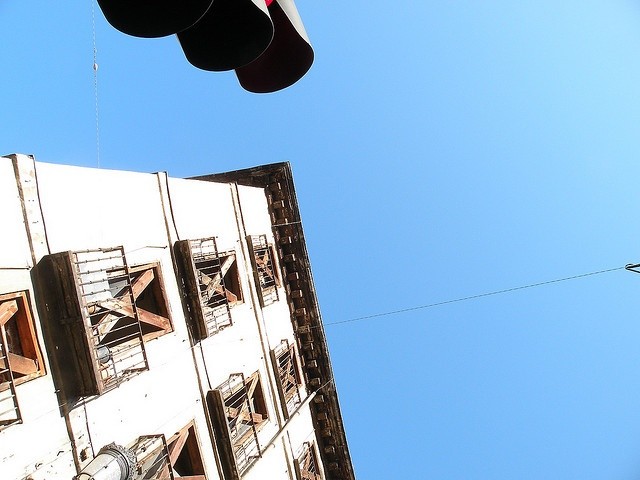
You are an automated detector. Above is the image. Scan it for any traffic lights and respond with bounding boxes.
[97,0,314,94]
